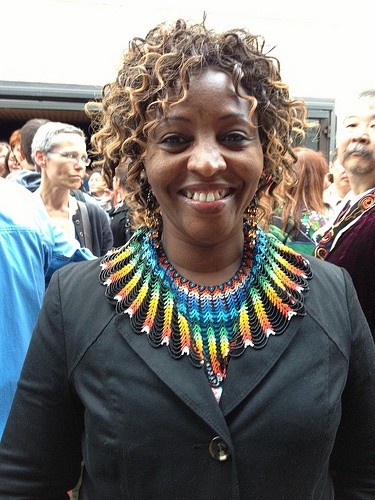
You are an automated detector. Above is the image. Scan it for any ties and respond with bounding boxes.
[315,201,349,259]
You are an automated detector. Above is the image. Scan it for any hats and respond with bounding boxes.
[10,130,22,145]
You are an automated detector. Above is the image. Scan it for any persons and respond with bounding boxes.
[0,176,99,441]
[1,20,375,500]
[312,90,375,343]
[2,119,133,258]
[268,147,355,255]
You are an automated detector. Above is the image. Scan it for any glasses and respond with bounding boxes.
[51,150,91,167]
